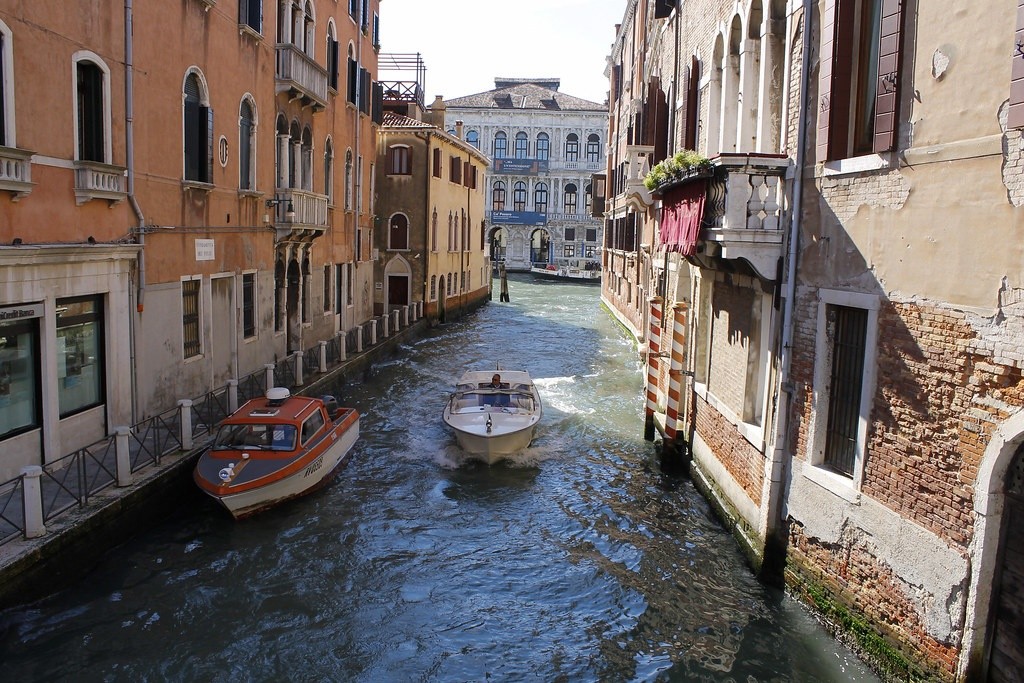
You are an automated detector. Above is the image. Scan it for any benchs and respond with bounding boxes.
[455,398,534,409]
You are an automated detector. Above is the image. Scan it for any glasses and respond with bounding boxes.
[492,378,499,381]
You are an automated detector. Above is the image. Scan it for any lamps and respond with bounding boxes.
[266,198,296,217]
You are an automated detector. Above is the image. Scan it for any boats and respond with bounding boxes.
[441,360,542,466]
[530,265,601,284]
[190,387,359,528]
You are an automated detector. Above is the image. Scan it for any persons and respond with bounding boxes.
[483,374,510,404]
